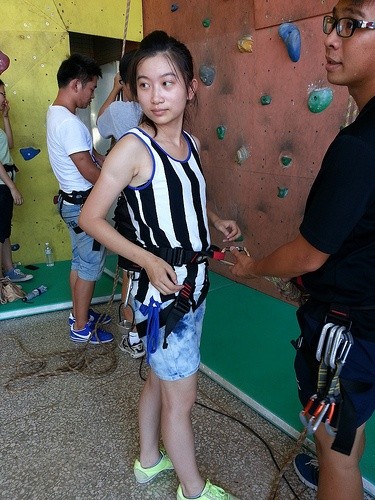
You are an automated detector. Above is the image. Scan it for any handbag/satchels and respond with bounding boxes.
[0,276,26,304]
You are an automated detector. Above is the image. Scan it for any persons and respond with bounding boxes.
[47,54,114,344]
[78,30,242,500]
[96,48,145,359]
[0,79,34,289]
[228,0,375,500]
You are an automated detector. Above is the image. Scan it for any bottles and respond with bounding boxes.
[44,242,54,267]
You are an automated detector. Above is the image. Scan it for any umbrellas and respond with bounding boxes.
[22,285,47,303]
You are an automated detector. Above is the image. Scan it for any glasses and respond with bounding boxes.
[323,16,375,38]
[119,80,124,85]
[0,92,6,98]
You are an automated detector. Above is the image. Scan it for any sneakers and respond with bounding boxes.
[117,331,145,359]
[68,308,112,327]
[3,268,33,282]
[134,442,175,484]
[69,320,114,344]
[176,479,242,500]
[293,453,319,492]
